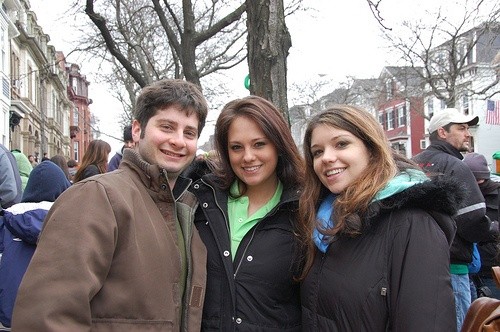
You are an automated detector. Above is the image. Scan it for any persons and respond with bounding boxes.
[0,127,137,331]
[11,79,209,332]
[292,103,472,332]
[190,96,305,332]
[402,108,500,332]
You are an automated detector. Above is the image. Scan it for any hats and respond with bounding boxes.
[461,152,490,180]
[428,108,479,134]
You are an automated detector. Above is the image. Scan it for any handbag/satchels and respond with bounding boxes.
[460,266,500,332]
